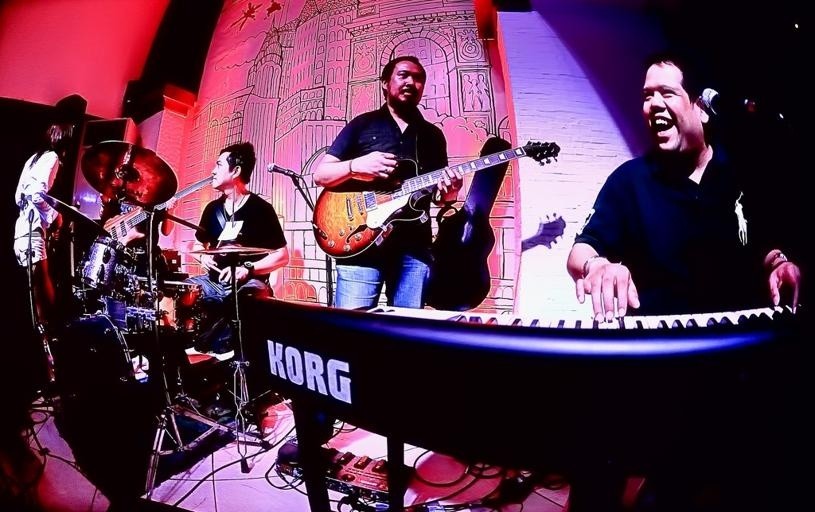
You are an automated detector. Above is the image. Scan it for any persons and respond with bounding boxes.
[277,55,463,461]
[13,120,82,381]
[193,141,290,433]
[99,191,179,281]
[565,58,802,511]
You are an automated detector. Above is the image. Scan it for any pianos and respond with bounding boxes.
[239,292,815,492]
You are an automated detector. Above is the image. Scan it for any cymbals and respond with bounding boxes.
[82,141,177,208]
[132,276,200,287]
[39,190,111,237]
[190,245,269,255]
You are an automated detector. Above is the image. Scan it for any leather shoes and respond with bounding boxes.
[278,434,329,461]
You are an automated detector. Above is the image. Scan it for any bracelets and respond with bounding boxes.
[767,253,789,271]
[582,255,600,277]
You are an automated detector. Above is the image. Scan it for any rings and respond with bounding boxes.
[385,167,389,174]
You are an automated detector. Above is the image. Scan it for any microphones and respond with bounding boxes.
[267,163,300,178]
[702,88,786,119]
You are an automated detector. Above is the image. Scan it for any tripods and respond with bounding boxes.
[140,212,274,504]
[216,251,266,474]
[30,215,115,428]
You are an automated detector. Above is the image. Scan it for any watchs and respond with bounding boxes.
[242,260,255,274]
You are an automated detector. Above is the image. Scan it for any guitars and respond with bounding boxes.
[312,140,561,260]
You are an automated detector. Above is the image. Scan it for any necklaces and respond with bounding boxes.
[223,191,248,222]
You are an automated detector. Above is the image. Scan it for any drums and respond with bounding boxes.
[78,235,142,298]
[103,291,160,331]
[52,314,145,484]
[129,276,208,335]
